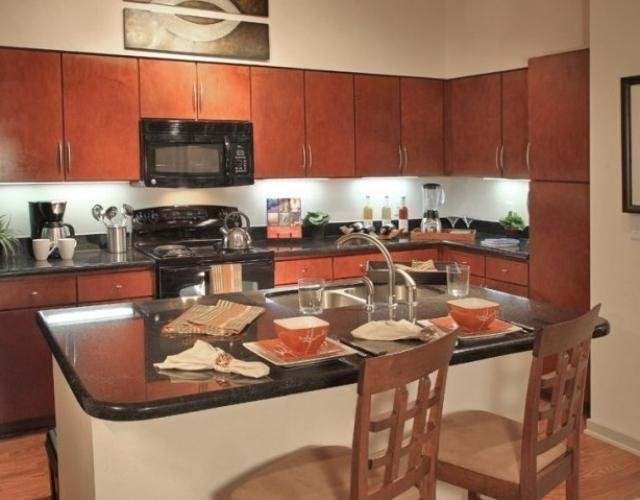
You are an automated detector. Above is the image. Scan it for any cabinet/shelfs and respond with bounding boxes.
[269,242,438,287]
[140,54,250,121]
[355,72,446,177]
[1,47,140,183]
[250,61,355,177]
[441,229,528,298]
[0,271,76,427]
[528,181,591,302]
[77,269,156,301]
[527,47,590,180]
[448,67,529,179]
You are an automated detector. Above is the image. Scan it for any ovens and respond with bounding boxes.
[158,260,275,301]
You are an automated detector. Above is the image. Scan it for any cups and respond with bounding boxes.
[58,239,78,261]
[104,253,128,267]
[106,226,128,255]
[59,259,76,268]
[31,238,55,261]
[446,264,470,296]
[297,276,325,315]
[33,260,53,269]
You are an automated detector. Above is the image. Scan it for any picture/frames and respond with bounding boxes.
[621,75,640,213]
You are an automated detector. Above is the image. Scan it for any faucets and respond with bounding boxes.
[334,234,397,308]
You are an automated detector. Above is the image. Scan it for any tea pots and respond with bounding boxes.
[217,211,254,252]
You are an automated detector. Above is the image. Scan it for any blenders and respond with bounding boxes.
[421,183,446,233]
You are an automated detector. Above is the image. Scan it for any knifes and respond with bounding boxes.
[504,316,535,333]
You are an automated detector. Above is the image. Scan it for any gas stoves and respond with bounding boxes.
[138,295,264,383]
[135,237,274,263]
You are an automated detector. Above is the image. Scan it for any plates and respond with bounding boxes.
[416,317,524,345]
[243,337,358,368]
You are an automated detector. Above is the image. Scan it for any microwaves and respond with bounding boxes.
[139,117,254,190]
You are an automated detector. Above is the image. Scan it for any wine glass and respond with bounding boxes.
[446,214,473,230]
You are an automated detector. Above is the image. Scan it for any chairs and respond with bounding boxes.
[414,303,599,500]
[231,328,459,499]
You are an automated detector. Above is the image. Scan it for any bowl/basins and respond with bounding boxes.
[270,315,332,353]
[447,297,499,332]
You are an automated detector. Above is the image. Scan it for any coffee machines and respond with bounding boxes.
[28,200,75,260]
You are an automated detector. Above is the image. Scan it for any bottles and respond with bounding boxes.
[363,196,374,228]
[398,195,410,234]
[382,196,392,227]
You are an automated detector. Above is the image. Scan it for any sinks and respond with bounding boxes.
[266,289,364,311]
[344,281,444,304]
